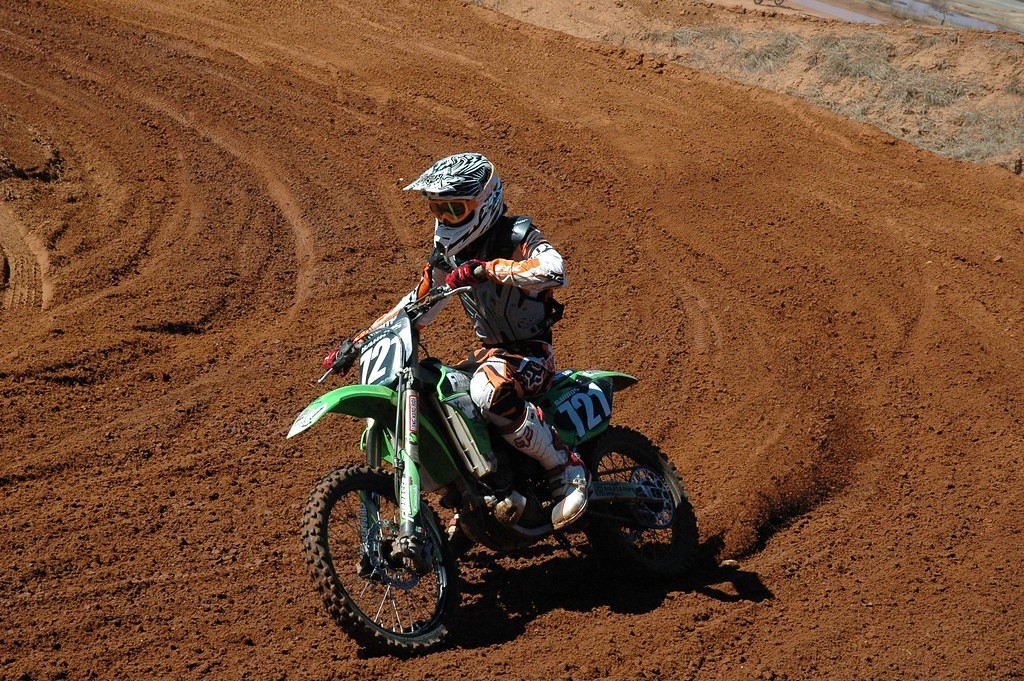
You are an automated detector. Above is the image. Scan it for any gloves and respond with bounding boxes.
[446,259,486,289]
[323,341,360,377]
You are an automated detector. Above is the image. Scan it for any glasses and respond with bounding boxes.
[428,198,472,224]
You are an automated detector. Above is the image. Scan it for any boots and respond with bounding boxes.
[502,401,592,530]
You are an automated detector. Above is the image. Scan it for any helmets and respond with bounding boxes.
[403,153,502,257]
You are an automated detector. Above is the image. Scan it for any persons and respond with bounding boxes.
[326,152,590,529]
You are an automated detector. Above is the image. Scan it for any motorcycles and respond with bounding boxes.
[283,266,701,661]
